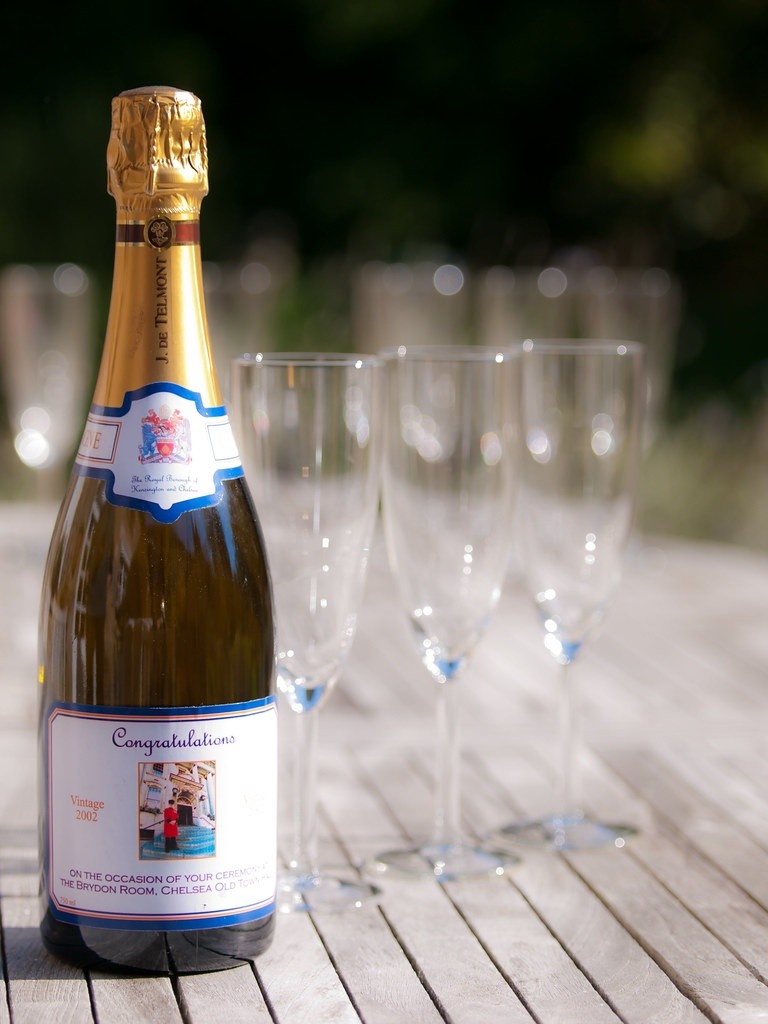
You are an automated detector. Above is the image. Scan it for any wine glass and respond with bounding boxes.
[504,341,643,862]
[374,348,519,882]
[238,350,380,915]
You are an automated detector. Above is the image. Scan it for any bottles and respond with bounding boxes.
[40,86,274,976]
